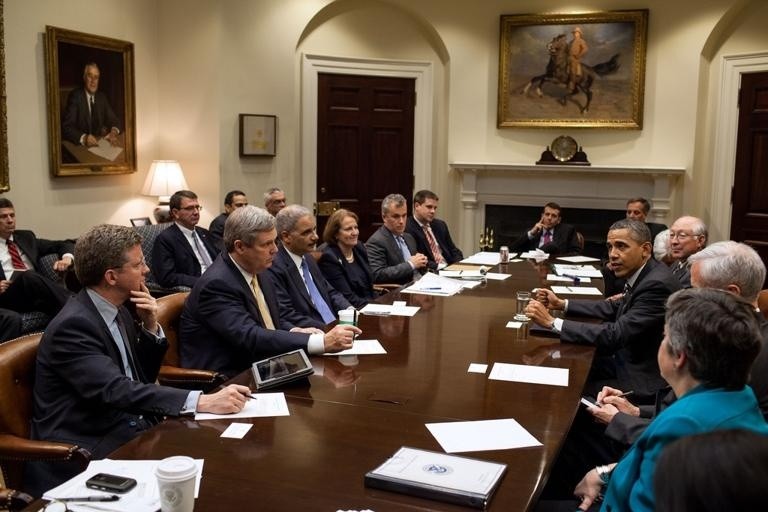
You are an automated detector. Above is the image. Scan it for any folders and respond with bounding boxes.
[363,445,508,511]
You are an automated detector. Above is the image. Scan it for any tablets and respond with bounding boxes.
[251,349,314,390]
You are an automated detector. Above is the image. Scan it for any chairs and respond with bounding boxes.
[133,221,193,298]
[0,488,33,512]
[757,288,768,322]
[372,283,403,288]
[1,332,229,488]
[21,237,75,334]
[309,251,390,311]
[155,289,229,392]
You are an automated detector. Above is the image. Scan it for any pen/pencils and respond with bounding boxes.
[563,274,580,282]
[57,495,120,503]
[353,310,356,341]
[220,385,253,398]
[460,270,463,274]
[596,390,634,406]
[430,288,441,290]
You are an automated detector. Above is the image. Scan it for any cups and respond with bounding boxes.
[338,310,359,338]
[500,246,510,264]
[514,291,535,322]
[154,455,199,511]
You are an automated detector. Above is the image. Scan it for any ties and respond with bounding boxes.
[397,235,411,262]
[114,313,139,380]
[544,230,550,245]
[423,226,442,265]
[192,232,211,267]
[6,240,27,269]
[301,259,336,324]
[252,276,275,329]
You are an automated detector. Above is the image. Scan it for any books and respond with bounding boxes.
[439,265,488,279]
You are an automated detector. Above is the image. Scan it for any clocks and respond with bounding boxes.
[535,135,591,166]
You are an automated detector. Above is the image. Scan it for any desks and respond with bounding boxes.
[16,251,607,512]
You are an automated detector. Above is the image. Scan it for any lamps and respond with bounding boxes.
[140,160,190,224]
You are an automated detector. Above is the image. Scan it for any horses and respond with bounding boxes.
[520,34,622,117]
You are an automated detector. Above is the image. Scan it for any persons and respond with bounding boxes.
[364,193,428,295]
[151,190,286,289]
[1,309,22,343]
[1,197,75,314]
[180,205,363,379]
[509,197,768,512]
[565,28,589,93]
[61,62,123,147]
[31,224,253,478]
[257,205,381,332]
[404,190,463,275]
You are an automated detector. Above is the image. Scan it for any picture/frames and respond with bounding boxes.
[497,9,650,130]
[0,0,10,193]
[239,112,277,157]
[130,217,153,226]
[45,24,138,178]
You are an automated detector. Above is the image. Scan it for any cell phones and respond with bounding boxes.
[86,473,137,493]
